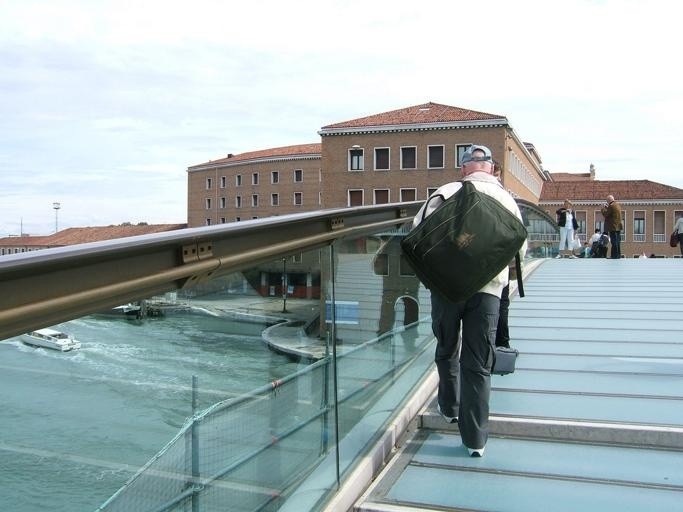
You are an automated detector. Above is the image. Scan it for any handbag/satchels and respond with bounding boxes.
[398,179,532,306]
[669,229,679,248]
[573,236,582,250]
[490,345,519,376]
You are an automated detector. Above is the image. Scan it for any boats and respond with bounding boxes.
[112,305,143,313]
[20,328,81,352]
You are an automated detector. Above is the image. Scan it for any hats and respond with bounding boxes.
[460,143,493,165]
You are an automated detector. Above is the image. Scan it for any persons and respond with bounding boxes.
[560,202,576,258]
[490,159,513,349]
[600,193,623,260]
[371,222,422,353]
[554,197,579,260]
[669,212,682,258]
[638,251,647,259]
[577,227,609,259]
[408,141,529,459]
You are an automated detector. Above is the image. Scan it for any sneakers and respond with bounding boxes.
[372,332,418,351]
[466,443,486,457]
[435,400,458,424]
[555,253,578,259]
